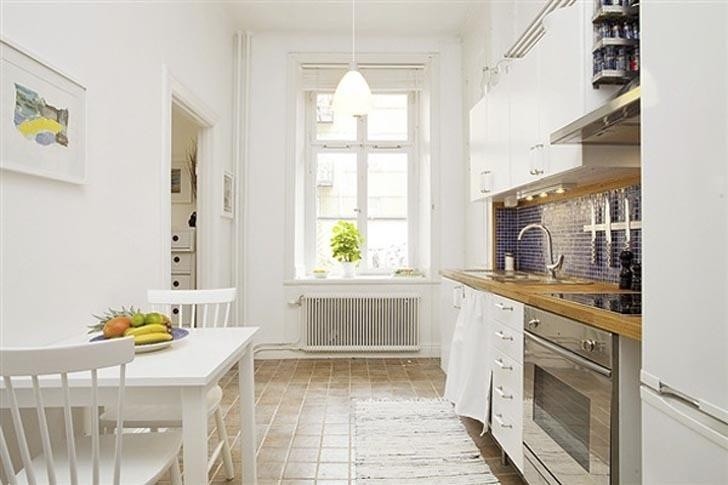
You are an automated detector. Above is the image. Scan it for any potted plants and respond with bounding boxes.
[330,218,363,277]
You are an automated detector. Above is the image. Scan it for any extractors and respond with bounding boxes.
[552,73,640,147]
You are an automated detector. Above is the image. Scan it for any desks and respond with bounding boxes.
[0,325,261,485]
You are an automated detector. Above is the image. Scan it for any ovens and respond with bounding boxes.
[521,330,617,485]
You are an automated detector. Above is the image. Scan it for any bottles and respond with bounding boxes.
[597,0,629,7]
[504,249,515,271]
[480,66,489,97]
[591,44,639,72]
[619,250,634,289]
[593,20,640,40]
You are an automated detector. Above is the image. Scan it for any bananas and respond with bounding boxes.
[124,324,173,336]
[134,332,172,345]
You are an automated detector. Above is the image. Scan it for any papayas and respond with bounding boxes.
[103,316,131,338]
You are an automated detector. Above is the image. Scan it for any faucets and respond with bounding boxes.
[515,222,565,282]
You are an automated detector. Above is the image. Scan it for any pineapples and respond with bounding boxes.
[86,305,173,335]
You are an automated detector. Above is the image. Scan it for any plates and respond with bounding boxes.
[89,327,190,354]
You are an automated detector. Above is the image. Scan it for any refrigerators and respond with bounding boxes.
[640,0,728,484]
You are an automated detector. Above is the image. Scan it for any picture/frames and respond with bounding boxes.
[220,168,235,218]
[171,159,193,204]
[0,36,89,189]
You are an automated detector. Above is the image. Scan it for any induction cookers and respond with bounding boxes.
[523,292,642,369]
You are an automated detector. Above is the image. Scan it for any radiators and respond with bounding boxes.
[298,296,421,353]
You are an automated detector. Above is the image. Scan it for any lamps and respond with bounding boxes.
[328,0,376,106]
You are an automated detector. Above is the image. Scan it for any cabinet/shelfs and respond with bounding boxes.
[170,228,197,325]
[471,0,641,206]
[443,269,641,476]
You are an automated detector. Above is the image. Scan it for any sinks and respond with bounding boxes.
[461,267,566,286]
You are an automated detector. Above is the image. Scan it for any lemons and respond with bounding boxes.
[314,270,325,273]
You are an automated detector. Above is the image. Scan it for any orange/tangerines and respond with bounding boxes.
[130,313,145,327]
[145,313,162,325]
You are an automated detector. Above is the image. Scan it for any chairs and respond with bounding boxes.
[98,286,239,481]
[1,334,185,485]
